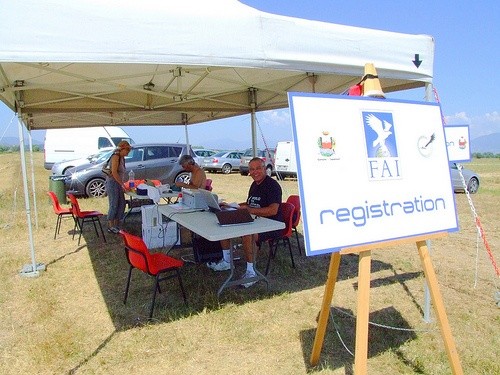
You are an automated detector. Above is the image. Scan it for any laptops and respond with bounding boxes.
[216,208,257,226]
[198,188,238,212]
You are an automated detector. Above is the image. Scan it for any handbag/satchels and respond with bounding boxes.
[102,155,120,176]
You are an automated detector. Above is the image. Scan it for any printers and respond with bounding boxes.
[181,189,218,208]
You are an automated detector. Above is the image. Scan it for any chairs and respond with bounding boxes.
[257,195,302,276]
[47,192,82,239]
[119,230,187,320]
[68,194,106,246]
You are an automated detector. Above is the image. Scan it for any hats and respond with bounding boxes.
[118,140,133,149]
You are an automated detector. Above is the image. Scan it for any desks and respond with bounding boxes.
[123,191,177,224]
[158,205,286,297]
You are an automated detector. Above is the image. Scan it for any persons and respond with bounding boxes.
[106,140,131,233]
[175,155,206,190]
[213,157,282,287]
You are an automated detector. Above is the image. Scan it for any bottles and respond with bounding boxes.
[178,194,183,205]
[129,169,135,194]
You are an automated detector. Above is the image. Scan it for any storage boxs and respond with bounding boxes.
[141,205,180,249]
[183,191,218,208]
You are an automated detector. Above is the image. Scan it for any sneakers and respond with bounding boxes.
[214,258,236,271]
[241,274,257,287]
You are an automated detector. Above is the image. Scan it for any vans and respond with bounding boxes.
[273,142,300,181]
[42,126,135,170]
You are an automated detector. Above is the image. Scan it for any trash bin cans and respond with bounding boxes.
[49,175,68,205]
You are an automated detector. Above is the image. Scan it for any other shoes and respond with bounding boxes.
[107,225,125,233]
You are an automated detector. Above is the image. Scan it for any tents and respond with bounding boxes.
[0,0,434,323]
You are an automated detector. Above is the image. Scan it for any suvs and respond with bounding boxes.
[49,143,202,198]
[196,148,272,176]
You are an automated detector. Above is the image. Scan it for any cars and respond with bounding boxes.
[448,163,481,195]
